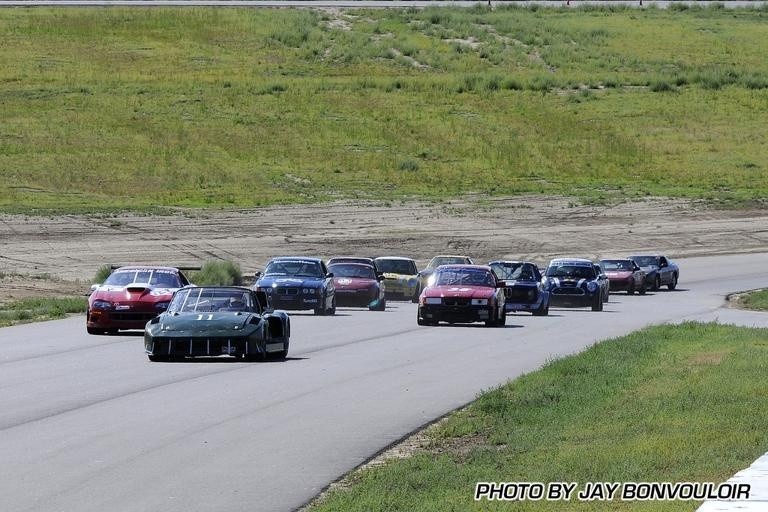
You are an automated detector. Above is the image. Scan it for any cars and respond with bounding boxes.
[628,255,679,291]
[417,263,507,326]
[542,257,609,311]
[420,254,475,287]
[251,256,336,315]
[599,258,647,295]
[327,256,387,311]
[375,257,421,303]
[489,260,551,315]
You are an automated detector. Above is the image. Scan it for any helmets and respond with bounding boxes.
[472,271,486,282]
[521,266,533,278]
[230,295,246,310]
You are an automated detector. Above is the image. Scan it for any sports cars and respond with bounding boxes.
[86,265,196,334]
[144,286,290,362]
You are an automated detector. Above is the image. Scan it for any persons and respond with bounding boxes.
[298,265,319,277]
[217,297,250,312]
[152,275,176,289]
[468,272,491,286]
